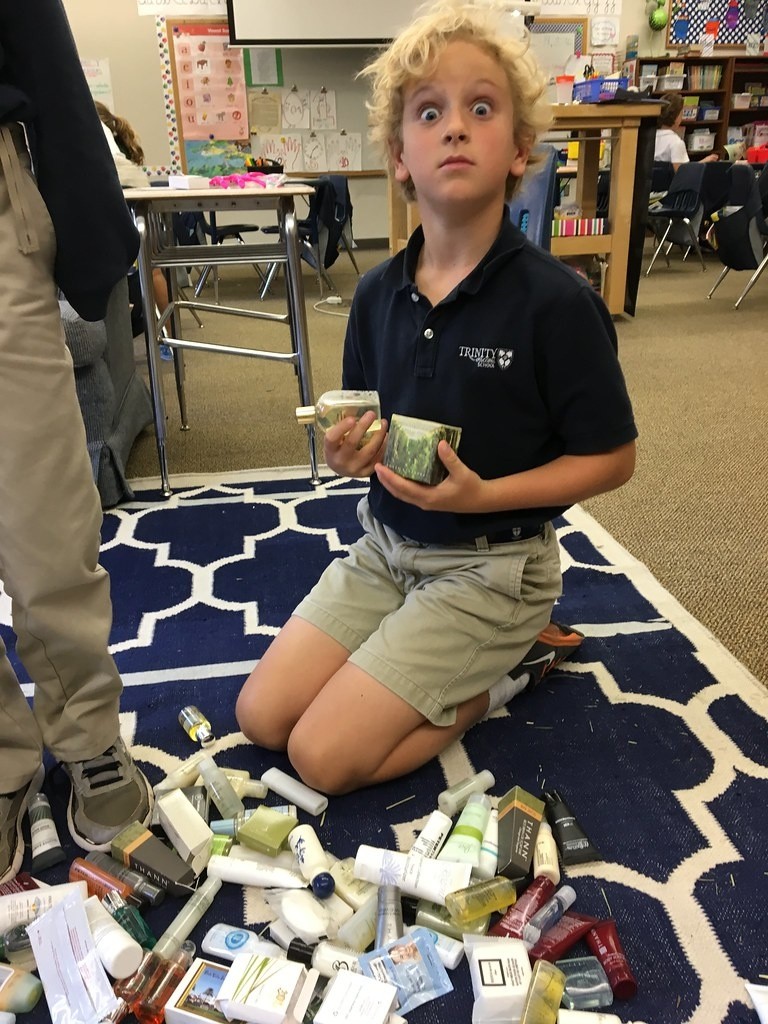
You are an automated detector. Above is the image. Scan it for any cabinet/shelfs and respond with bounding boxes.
[123,184,322,497]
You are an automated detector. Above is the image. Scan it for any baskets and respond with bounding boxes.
[572,75,631,103]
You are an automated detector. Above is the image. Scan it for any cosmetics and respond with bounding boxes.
[295,389,382,434]
[0,705,641,1024]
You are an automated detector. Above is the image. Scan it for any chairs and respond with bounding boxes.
[645,162,707,278]
[296,175,365,277]
[256,180,342,301]
[194,211,275,298]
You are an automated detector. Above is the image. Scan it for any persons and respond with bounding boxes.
[235,0,640,798]
[0,0,153,883]
[653,92,718,174]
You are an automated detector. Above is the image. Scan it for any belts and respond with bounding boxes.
[464,522,545,546]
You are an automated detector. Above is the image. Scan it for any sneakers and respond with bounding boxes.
[158,344,174,361]
[61,734,156,854]
[524,623,586,681]
[0,762,47,886]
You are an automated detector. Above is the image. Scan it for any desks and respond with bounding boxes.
[387,100,671,318]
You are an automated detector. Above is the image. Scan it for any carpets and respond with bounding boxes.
[0,464,768,1024]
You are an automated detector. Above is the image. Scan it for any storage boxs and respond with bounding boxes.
[574,77,628,101]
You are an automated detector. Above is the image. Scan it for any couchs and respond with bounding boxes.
[58,275,152,509]
